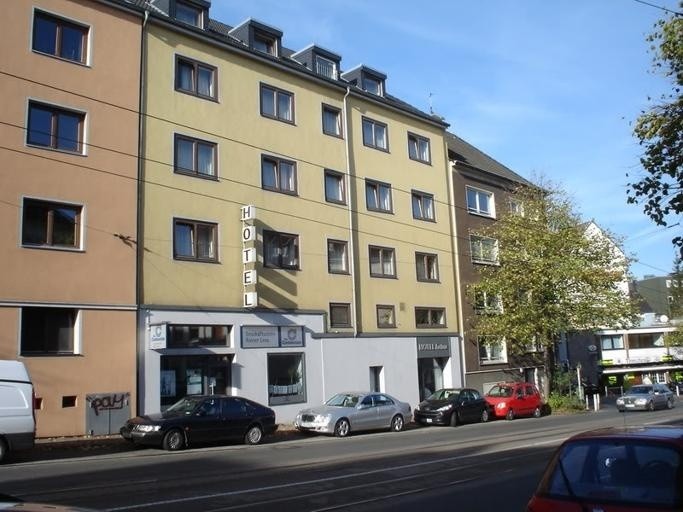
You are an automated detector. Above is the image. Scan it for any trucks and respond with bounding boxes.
[0,358,37,465]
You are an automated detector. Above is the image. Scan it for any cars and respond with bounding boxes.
[613,384,675,413]
[479,382,547,422]
[116,395,282,450]
[669,381,682,393]
[416,387,493,427]
[291,389,414,435]
[526,424,683,510]
[561,379,601,400]
